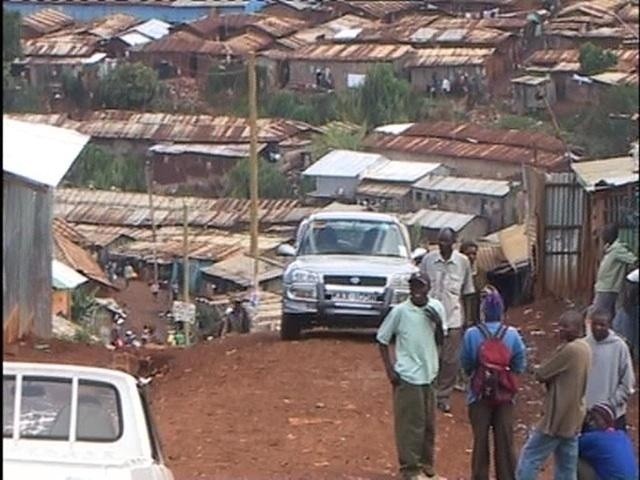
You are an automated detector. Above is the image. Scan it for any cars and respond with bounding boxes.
[2,360,174,480]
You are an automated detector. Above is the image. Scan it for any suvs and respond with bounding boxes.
[275,209,421,338]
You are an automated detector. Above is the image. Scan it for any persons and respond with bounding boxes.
[514,221,639,480]
[458,291,528,480]
[458,240,489,395]
[417,225,475,412]
[106,258,186,349]
[224,300,249,336]
[425,68,471,98]
[360,198,376,211]
[376,272,448,480]
[307,227,343,251]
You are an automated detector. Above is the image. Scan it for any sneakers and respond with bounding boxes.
[429,471,449,480]
[408,471,429,480]
[438,401,452,412]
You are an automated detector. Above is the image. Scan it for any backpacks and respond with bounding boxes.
[470,323,521,409]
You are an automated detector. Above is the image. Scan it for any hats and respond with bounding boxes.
[481,288,503,319]
[593,400,617,428]
[407,271,432,287]
